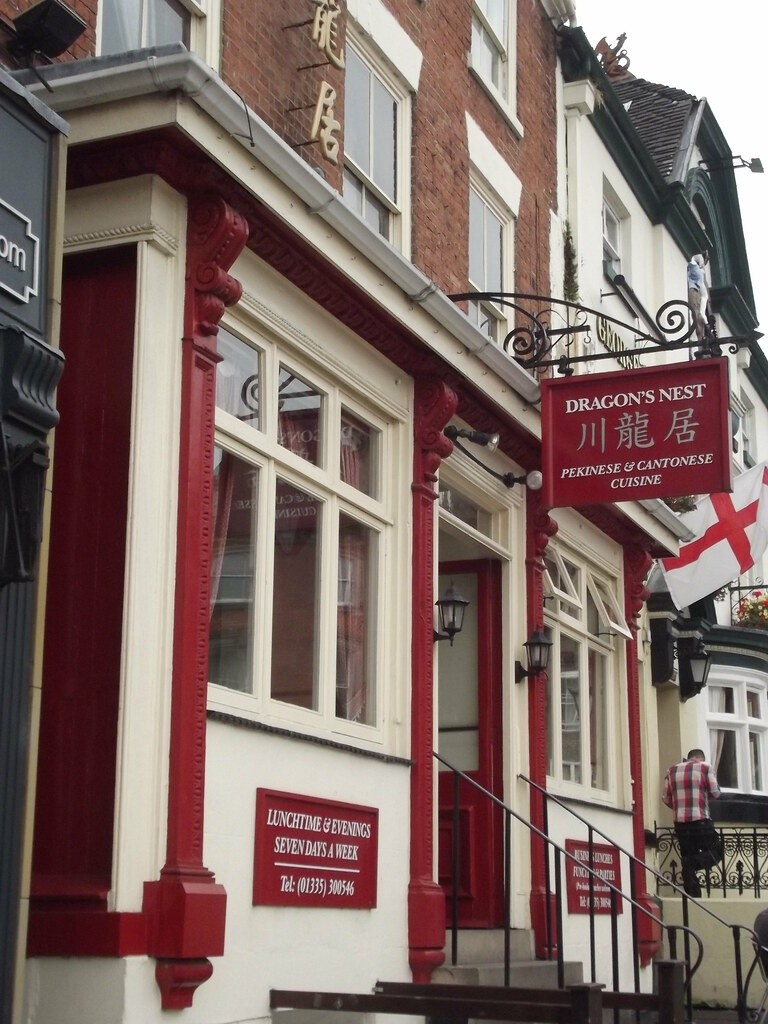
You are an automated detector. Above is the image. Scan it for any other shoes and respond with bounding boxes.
[681,856,695,883]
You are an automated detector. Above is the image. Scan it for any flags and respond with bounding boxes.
[658,461,768,611]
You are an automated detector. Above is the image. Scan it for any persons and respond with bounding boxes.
[662,749,723,899]
